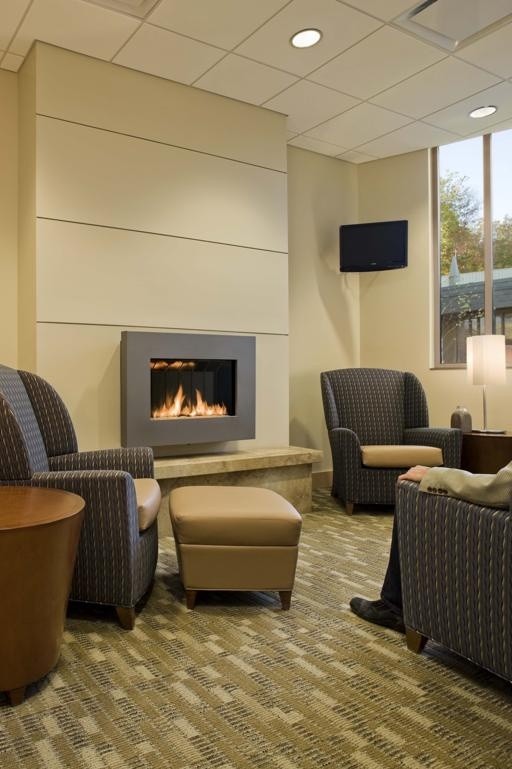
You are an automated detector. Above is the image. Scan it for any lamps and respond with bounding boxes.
[466,333,508,435]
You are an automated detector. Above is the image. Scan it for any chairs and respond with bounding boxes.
[394,479,510,686]
[1,366,165,631]
[319,368,464,517]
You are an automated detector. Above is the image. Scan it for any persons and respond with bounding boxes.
[348,459,512,634]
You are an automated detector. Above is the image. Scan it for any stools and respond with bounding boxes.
[168,485,304,611]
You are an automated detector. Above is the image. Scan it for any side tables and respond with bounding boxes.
[0,485,87,706]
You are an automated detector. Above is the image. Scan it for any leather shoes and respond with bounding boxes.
[350,597,406,634]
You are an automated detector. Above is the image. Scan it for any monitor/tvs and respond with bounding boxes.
[340,220,408,272]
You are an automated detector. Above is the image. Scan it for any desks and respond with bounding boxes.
[462,432,512,474]
